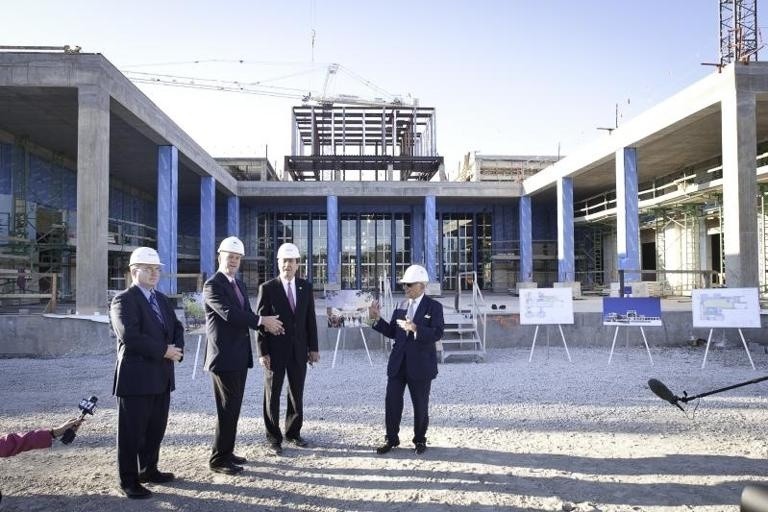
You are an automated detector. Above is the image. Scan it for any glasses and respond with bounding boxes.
[403,283,414,287]
[150,290,165,336]
[136,267,161,273]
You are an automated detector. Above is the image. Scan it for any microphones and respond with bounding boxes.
[62,394,100,444]
[647,378,681,410]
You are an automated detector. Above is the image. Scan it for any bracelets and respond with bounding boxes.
[48,428,55,438]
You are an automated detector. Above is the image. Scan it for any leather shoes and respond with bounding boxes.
[139,473,174,482]
[210,464,244,474]
[286,437,308,446]
[415,443,426,455]
[120,482,151,498]
[377,440,399,453]
[268,444,282,455]
[231,453,246,463]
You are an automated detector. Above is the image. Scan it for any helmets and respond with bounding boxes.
[129,247,166,266]
[276,242,301,258]
[217,236,246,256]
[397,264,429,284]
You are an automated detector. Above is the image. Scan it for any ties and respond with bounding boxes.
[231,280,245,314]
[288,282,295,315]
[407,300,416,321]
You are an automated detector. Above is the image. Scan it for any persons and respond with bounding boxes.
[255,243,321,454]
[108,245,185,499]
[367,265,446,455]
[0,416,78,457]
[202,236,285,474]
